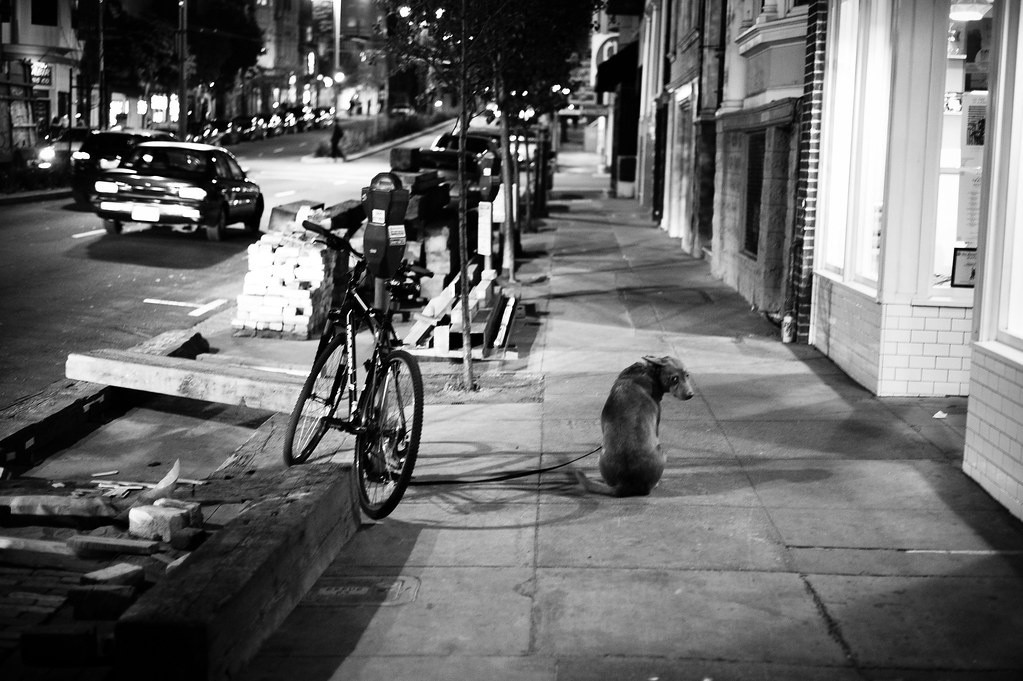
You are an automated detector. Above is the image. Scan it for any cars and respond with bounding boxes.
[183,107,336,148]
[38,128,93,182]
[88,140,264,242]
[72,130,176,215]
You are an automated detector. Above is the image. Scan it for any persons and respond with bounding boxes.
[43,117,63,144]
[330,119,344,157]
[113,113,129,129]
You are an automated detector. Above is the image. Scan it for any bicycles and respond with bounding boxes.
[282,219,435,522]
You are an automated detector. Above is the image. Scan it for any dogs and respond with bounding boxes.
[574,355,694,499]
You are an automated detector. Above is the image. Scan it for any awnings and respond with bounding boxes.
[596,42,638,91]
